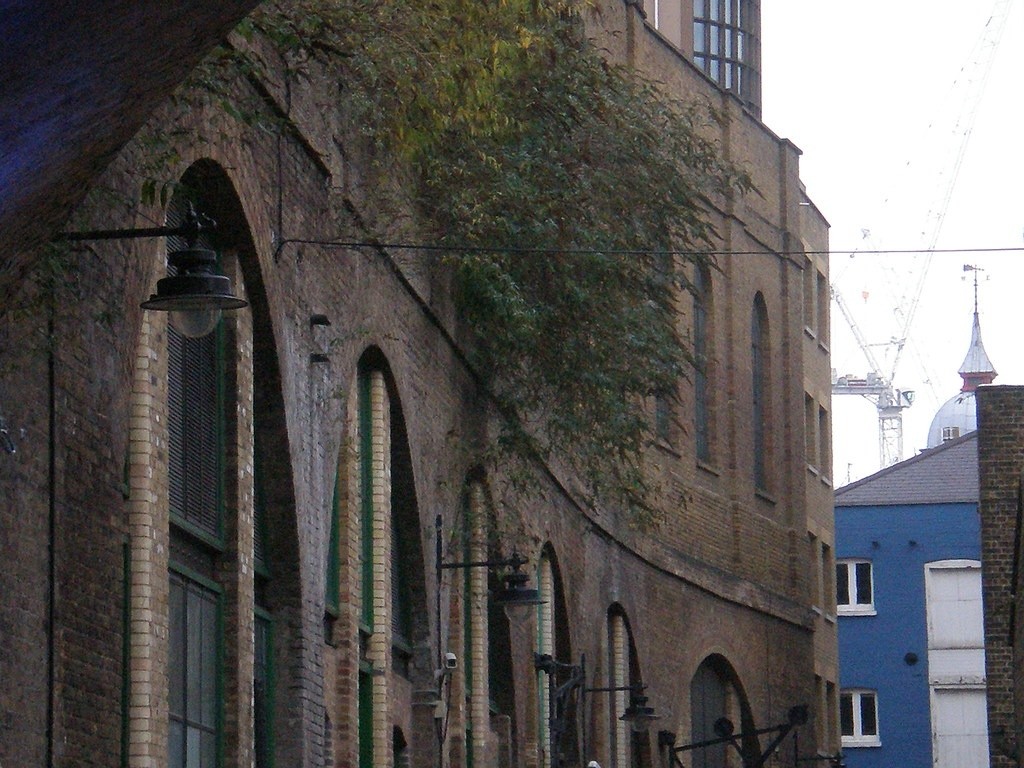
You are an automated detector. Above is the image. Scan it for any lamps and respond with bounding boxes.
[581,654,660,733]
[440,543,548,623]
[48,197,249,338]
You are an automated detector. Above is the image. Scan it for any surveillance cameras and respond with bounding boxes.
[444,653,457,669]
